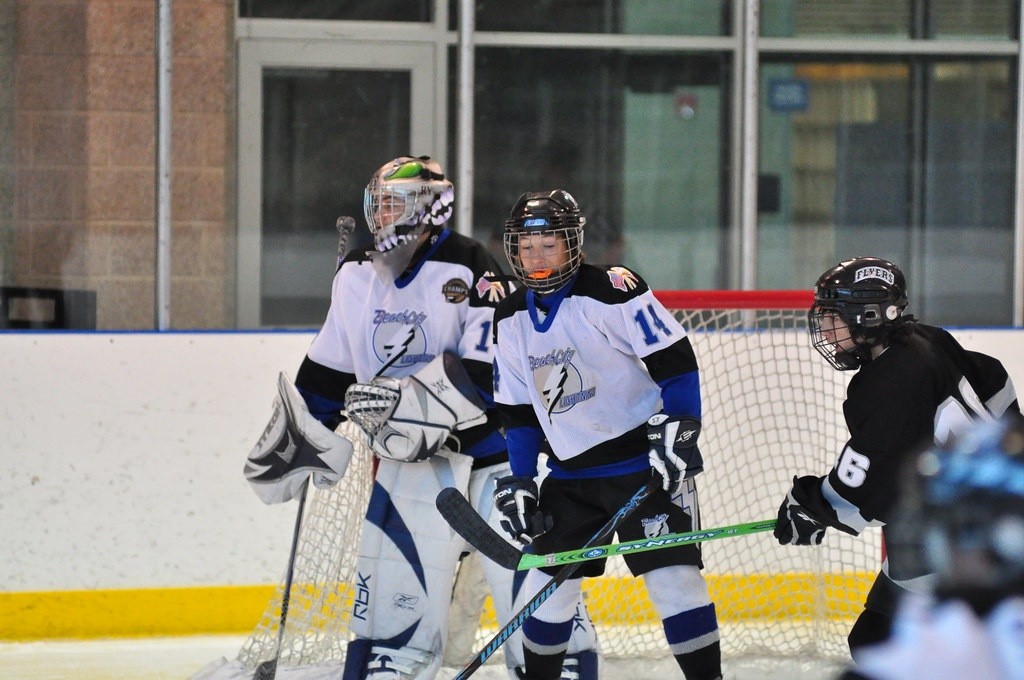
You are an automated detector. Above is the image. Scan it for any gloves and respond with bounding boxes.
[494,475,549,545]
[773,474,824,545]
[647,414,704,492]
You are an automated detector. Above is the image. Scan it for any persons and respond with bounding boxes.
[493,187,722,680]
[888,418,1024,679]
[243,154,599,679]
[772,255,1022,680]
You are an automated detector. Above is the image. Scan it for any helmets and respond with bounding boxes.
[506,190,585,293]
[816,257,907,368]
[371,157,455,253]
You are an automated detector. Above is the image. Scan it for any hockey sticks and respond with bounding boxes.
[249,468,313,680]
[429,476,787,577]
[435,470,662,680]
